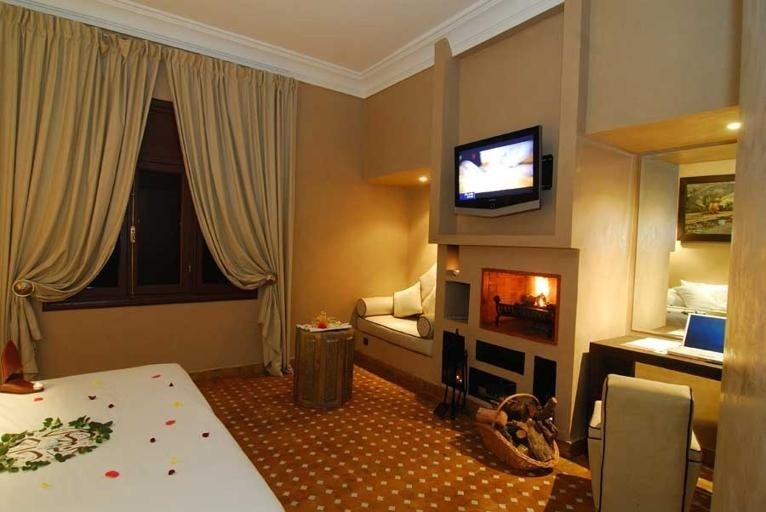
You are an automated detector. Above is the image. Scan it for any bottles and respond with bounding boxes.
[317,311,327,329]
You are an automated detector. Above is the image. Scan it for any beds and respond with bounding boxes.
[0,362,286,512]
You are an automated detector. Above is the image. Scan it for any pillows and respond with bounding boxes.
[392,262,436,319]
[667,279,727,311]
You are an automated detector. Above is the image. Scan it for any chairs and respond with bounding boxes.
[587,373,704,512]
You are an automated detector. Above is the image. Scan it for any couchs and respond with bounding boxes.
[353,293,435,382]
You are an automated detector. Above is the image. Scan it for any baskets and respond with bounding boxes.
[474,394,560,471]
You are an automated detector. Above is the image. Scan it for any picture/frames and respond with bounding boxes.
[676,174,736,241]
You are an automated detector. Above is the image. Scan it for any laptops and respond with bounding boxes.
[668,313,727,365]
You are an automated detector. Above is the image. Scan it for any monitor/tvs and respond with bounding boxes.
[453,124,542,218]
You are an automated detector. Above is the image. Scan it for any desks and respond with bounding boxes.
[584,333,722,456]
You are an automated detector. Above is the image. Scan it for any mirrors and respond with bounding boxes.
[630,139,738,339]
